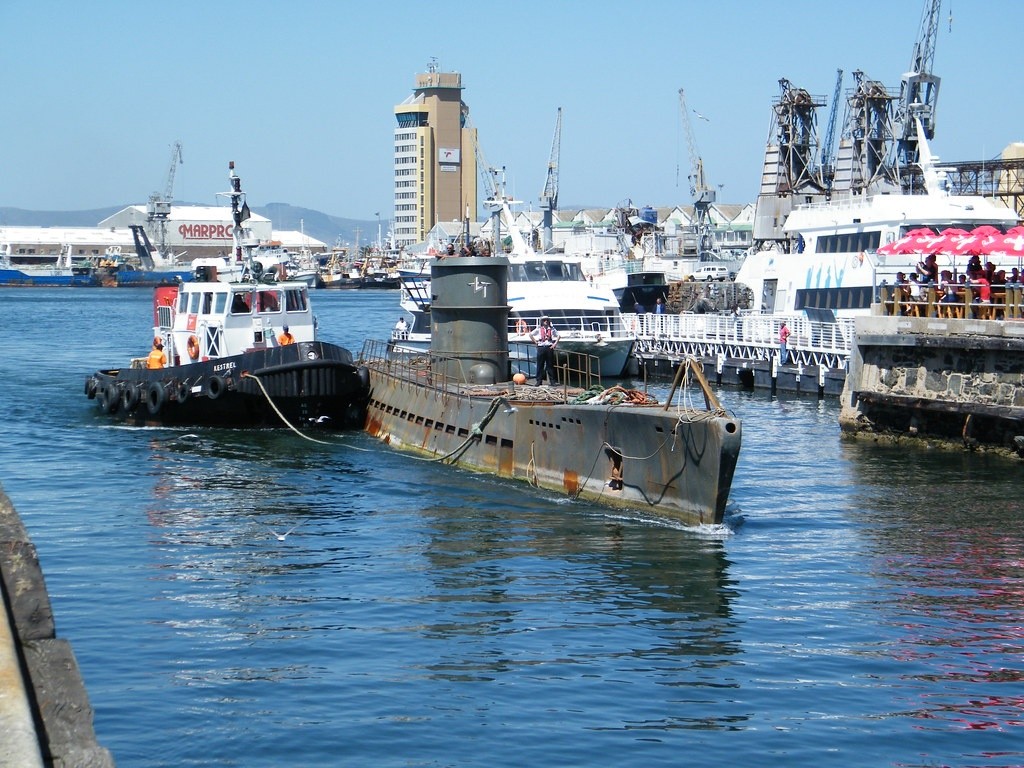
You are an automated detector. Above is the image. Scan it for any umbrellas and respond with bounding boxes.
[877,226,1024,282]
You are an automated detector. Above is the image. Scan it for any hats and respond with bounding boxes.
[282,324,288,330]
[156,344,164,348]
[539,315,550,321]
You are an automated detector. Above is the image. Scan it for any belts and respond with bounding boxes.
[539,346,551,347]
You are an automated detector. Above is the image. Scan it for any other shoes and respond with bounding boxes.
[550,382,557,386]
[535,381,542,386]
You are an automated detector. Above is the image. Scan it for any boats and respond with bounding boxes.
[84,278,358,420]
[1,226,403,291]
[358,257,741,531]
[384,0,1024,396]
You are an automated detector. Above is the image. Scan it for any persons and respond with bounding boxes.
[889,254,1024,320]
[434,241,491,260]
[146,344,166,369]
[633,302,644,314]
[278,324,294,346]
[395,317,408,340]
[529,316,560,387]
[779,321,790,365]
[652,298,667,314]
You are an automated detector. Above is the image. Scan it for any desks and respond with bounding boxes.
[935,287,1007,320]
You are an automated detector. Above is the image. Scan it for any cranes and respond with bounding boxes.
[457,103,501,254]
[538,106,563,252]
[676,87,720,260]
[146,142,183,258]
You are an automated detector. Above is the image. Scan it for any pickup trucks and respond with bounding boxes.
[687,266,728,282]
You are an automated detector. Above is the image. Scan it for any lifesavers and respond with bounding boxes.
[147,381,169,416]
[123,383,138,411]
[84,375,96,399]
[187,335,199,359]
[177,384,190,404]
[99,383,120,413]
[205,374,227,400]
[516,320,527,336]
